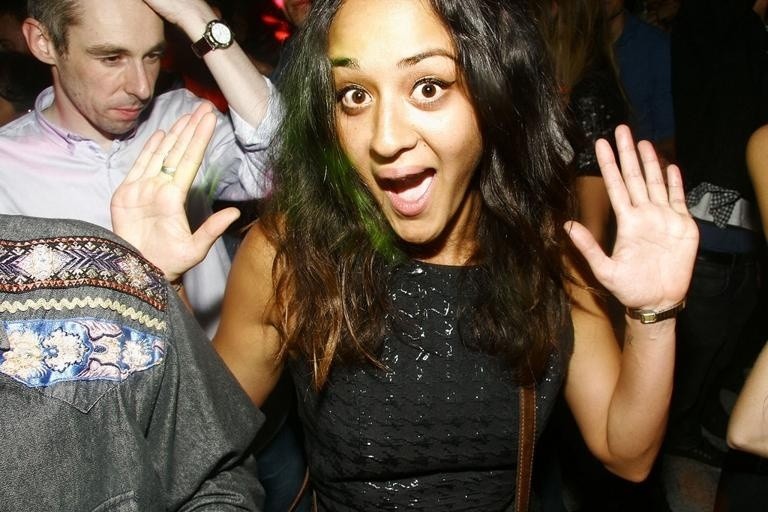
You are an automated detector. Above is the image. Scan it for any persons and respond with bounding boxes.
[1,0,766,512]
